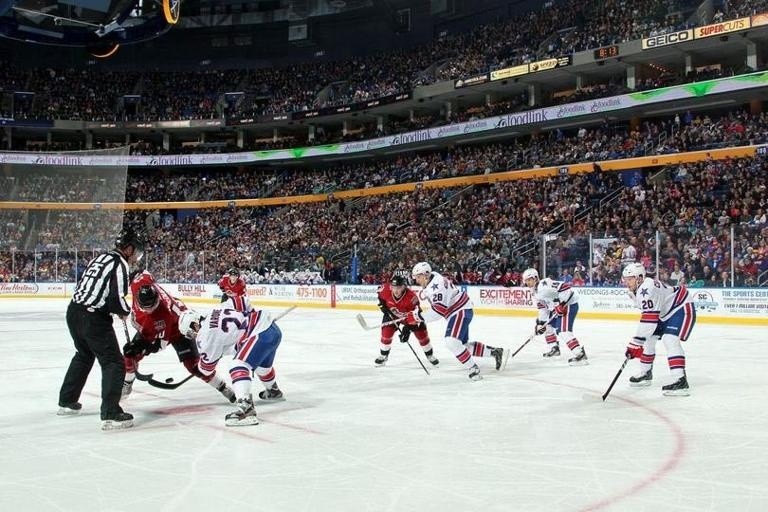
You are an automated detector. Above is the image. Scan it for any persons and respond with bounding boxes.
[177,294,284,421]
[1,1,768,288]
[621,263,696,391]
[123,269,236,404]
[55,230,145,431]
[522,268,590,362]
[216,269,247,303]
[374,276,439,367]
[411,261,505,379]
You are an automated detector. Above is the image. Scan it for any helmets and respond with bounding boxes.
[177,309,201,339]
[622,263,646,280]
[391,275,407,287]
[412,261,432,276]
[228,268,240,279]
[137,284,160,312]
[115,227,144,262]
[523,268,539,285]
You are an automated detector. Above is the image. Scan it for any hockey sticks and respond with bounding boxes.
[583,355,629,402]
[147,302,296,389]
[356,306,408,331]
[122,316,153,382]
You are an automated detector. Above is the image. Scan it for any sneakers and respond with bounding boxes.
[630,370,689,390]
[569,345,587,362]
[428,348,503,378]
[543,346,560,357]
[56,381,283,420]
[375,354,391,364]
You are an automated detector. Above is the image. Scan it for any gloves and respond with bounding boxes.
[398,325,409,342]
[555,304,568,316]
[406,311,415,326]
[625,344,644,359]
[123,340,140,358]
[535,323,546,335]
